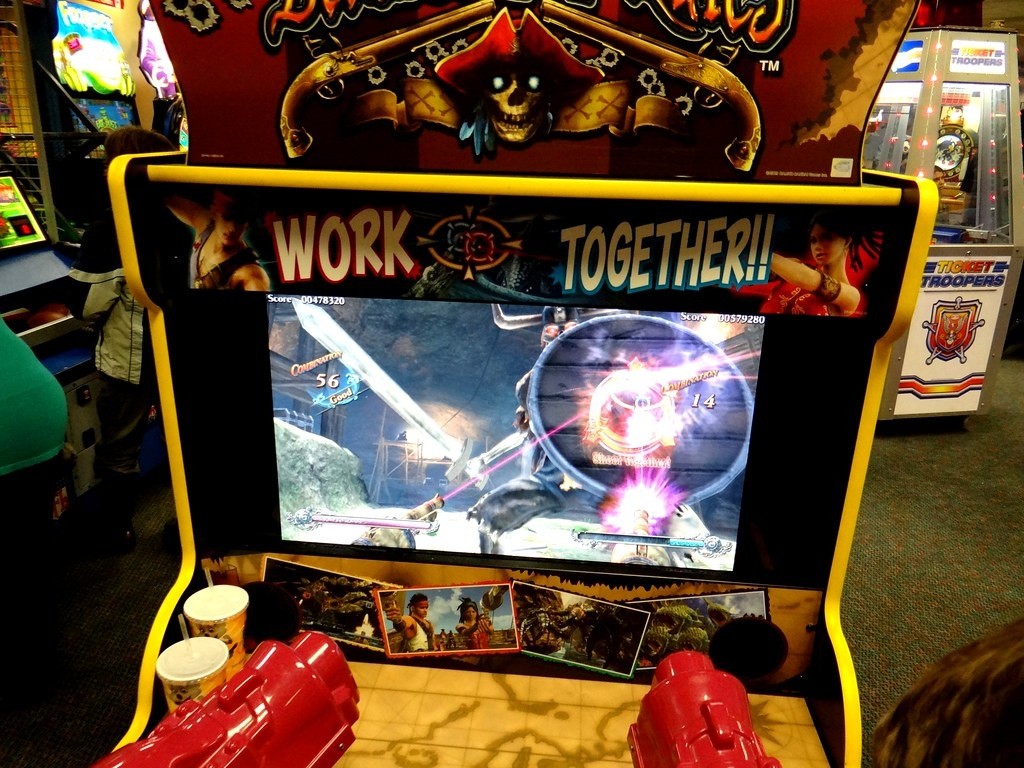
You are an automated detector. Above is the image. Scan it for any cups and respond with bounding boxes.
[183,585,249,676]
[155,636,229,713]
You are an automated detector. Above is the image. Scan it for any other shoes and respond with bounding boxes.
[92,524,135,557]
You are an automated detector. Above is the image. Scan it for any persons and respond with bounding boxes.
[63,126,183,555]
